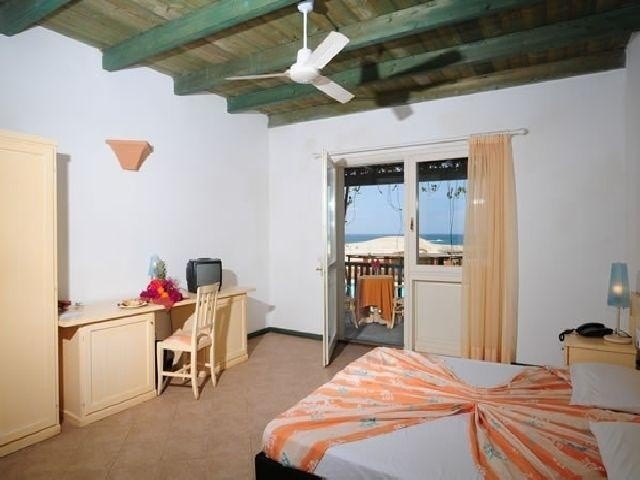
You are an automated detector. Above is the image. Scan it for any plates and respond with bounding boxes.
[112,300,148,308]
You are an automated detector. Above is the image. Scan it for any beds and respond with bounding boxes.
[254,345,640,480]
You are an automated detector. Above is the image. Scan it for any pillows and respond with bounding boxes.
[588,421,640,480]
[567,361,640,414]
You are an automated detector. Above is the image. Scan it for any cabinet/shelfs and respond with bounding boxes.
[0,129,61,458]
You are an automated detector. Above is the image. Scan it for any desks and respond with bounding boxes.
[59,287,256,428]
[355,275,394,330]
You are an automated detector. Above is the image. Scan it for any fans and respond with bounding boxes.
[223,0,356,106]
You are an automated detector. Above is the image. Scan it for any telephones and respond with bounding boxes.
[578,322,613,337]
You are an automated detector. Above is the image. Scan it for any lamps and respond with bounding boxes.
[603,262,633,343]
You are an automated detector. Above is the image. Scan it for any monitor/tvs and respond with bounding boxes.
[185,257,222,293]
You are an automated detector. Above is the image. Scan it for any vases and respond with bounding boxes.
[373,268,378,275]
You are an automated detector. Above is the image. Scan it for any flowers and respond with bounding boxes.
[370,258,381,270]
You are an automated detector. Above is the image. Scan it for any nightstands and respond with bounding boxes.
[563,332,639,370]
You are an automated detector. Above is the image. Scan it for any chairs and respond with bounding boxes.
[156,282,220,400]
[344,284,359,329]
[390,284,405,330]
